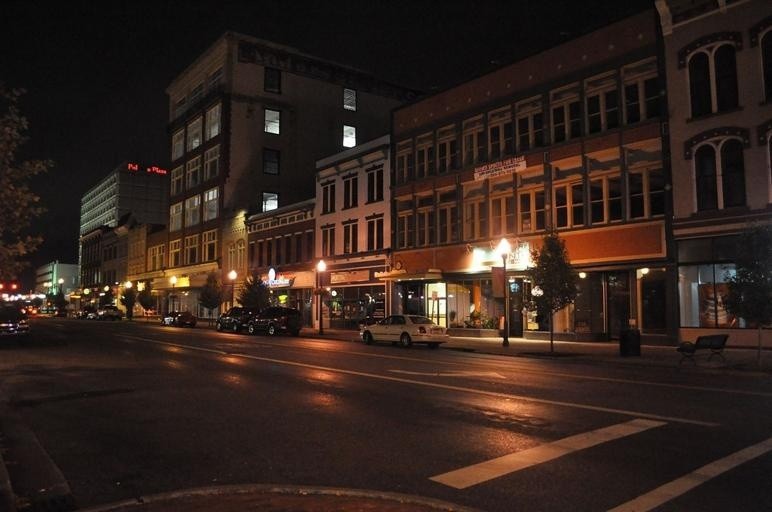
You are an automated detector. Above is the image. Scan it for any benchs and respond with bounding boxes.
[676,334,729,367]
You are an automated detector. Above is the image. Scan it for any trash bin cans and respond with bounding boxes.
[620,328,640,357]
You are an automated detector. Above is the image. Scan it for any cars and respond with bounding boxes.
[357,314,451,348]
[53,304,124,321]
[160,310,197,327]
[214,306,252,332]
[246,306,304,338]
[0,302,32,337]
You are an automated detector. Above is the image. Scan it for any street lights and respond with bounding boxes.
[58,277,65,292]
[499,234,511,348]
[170,274,178,312]
[43,281,54,314]
[315,257,327,335]
